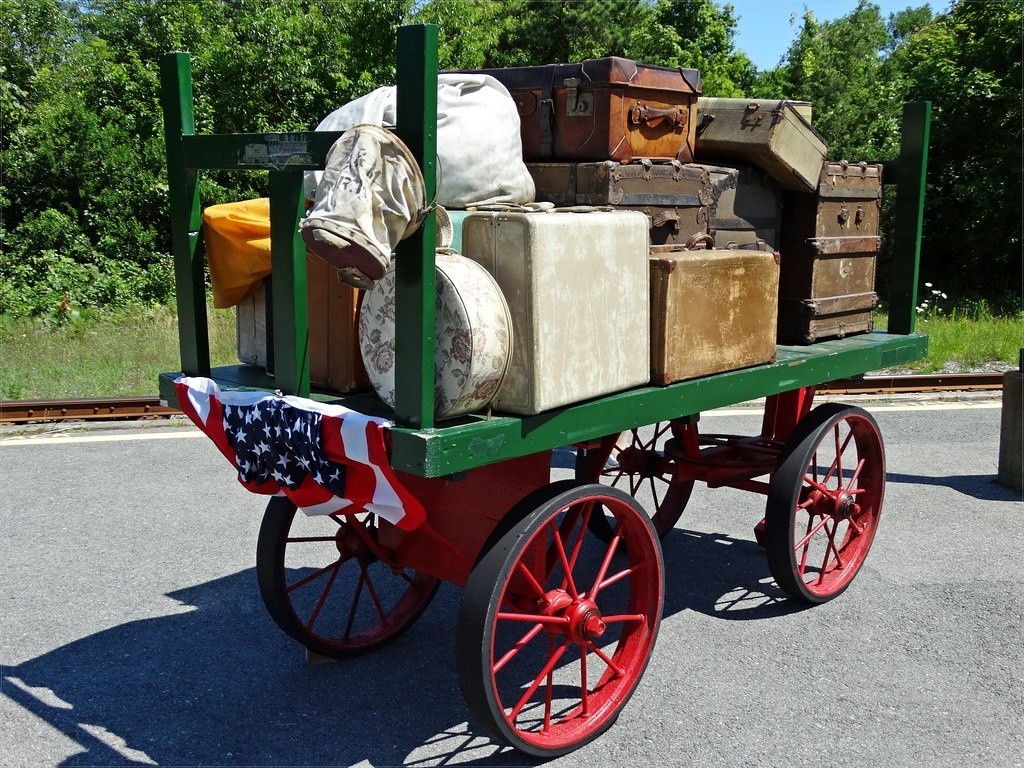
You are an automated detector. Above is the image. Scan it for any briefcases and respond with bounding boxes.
[463,205,652,418]
[237,271,273,372]
[305,249,374,393]
[437,56,704,166]
[650,233,783,390]
[695,97,882,344]
[524,156,714,250]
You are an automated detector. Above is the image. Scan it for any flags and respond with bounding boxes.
[172,375,426,532]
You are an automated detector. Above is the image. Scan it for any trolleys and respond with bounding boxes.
[156,23,936,764]
